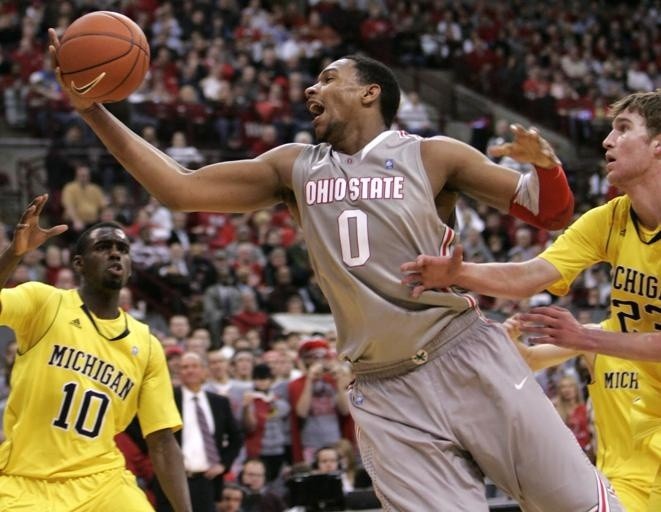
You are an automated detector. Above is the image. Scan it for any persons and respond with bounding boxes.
[1,340,18,402]
[399,92,661,512]
[1,2,661,320]
[118,316,382,511]
[1,192,192,511]
[539,357,598,465]
[48,28,622,512]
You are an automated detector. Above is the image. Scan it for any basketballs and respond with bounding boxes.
[57,11,150,104]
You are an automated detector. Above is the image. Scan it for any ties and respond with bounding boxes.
[192,395,221,466]
